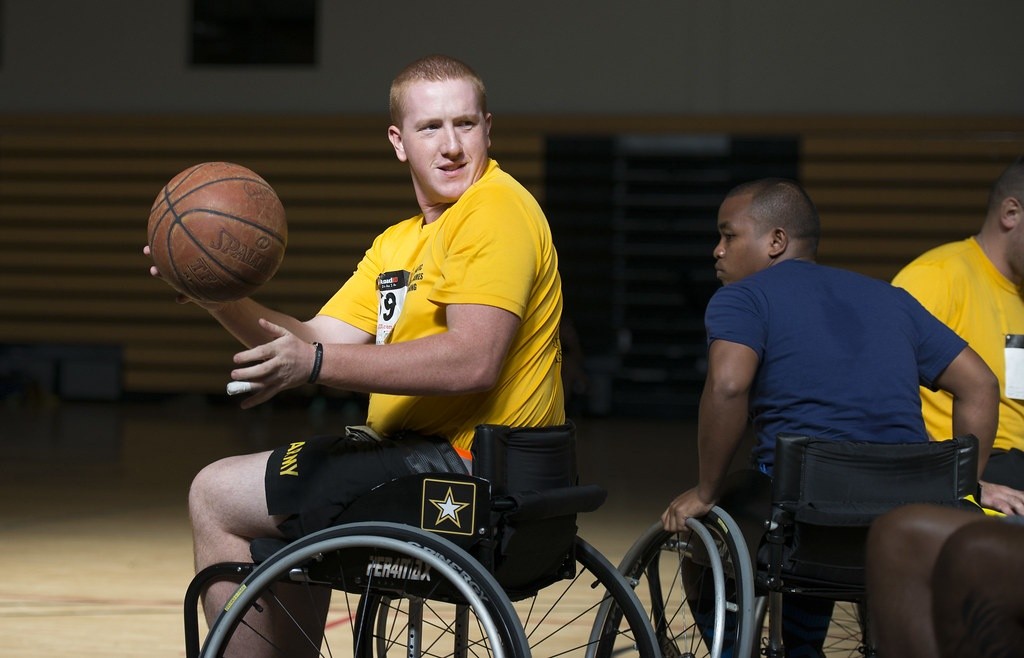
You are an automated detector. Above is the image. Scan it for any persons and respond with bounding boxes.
[888,145,1024,518]
[143,56,565,658]
[862,504,1024,658]
[659,176,999,658]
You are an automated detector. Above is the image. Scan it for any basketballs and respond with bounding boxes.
[150,162,287,302]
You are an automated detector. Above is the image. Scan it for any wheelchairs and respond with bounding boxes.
[184,415,663,658]
[586,433,982,658]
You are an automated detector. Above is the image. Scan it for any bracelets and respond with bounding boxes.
[308,341,324,384]
